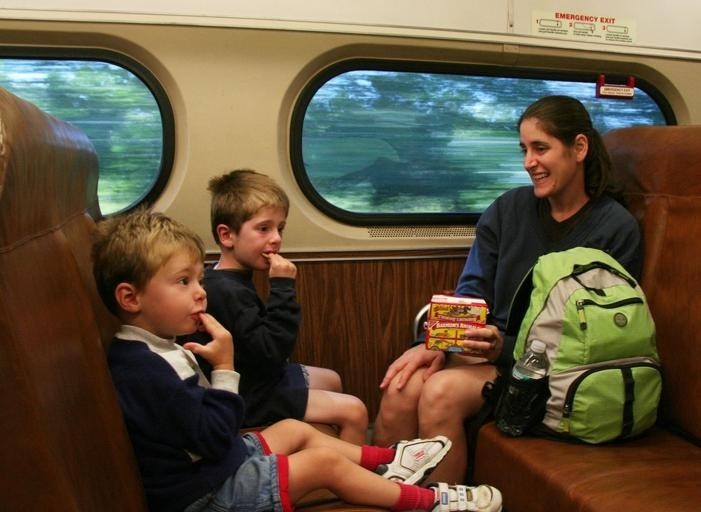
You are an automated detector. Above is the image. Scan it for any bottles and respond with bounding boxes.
[495,337,549,439]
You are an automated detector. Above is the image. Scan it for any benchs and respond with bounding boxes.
[0,87,388,512]
[462,124,676,510]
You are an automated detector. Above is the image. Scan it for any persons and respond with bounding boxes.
[372,95,641,489]
[90,212,503,512]
[184,169,369,447]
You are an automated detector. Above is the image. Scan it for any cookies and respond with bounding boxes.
[260,251,269,259]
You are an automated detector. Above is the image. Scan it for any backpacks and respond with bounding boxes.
[481,245,664,445]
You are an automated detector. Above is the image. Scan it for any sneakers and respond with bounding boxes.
[375,436,451,486]
[426,480,503,512]
